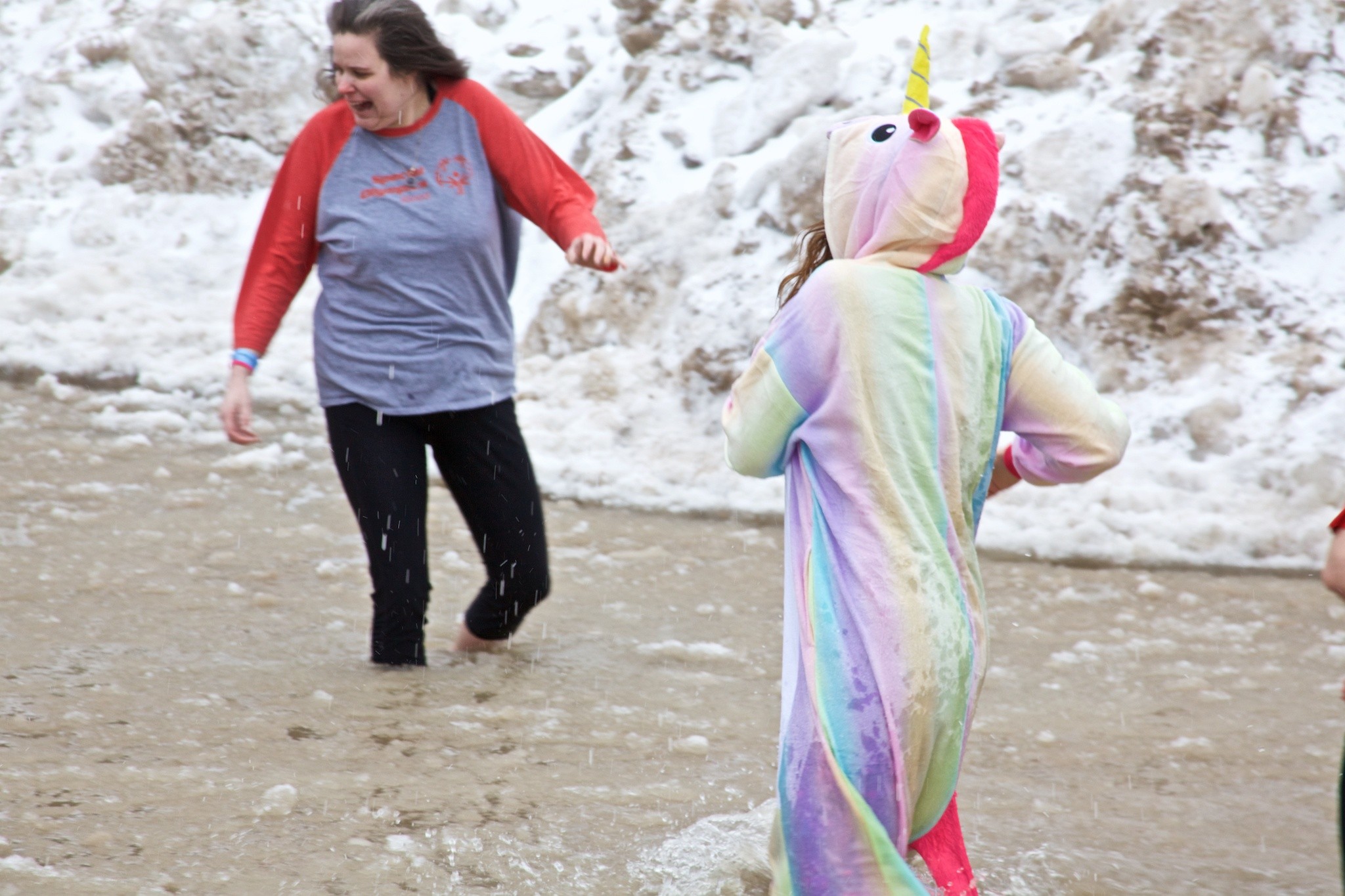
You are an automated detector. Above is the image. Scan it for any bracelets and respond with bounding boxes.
[232,353,256,369]
[232,359,252,374]
[236,349,257,361]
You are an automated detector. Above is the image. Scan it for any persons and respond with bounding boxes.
[1323,505,1345,896]
[217,1,618,666]
[723,21,1131,896]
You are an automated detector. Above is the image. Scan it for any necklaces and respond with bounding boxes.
[383,103,424,187]
